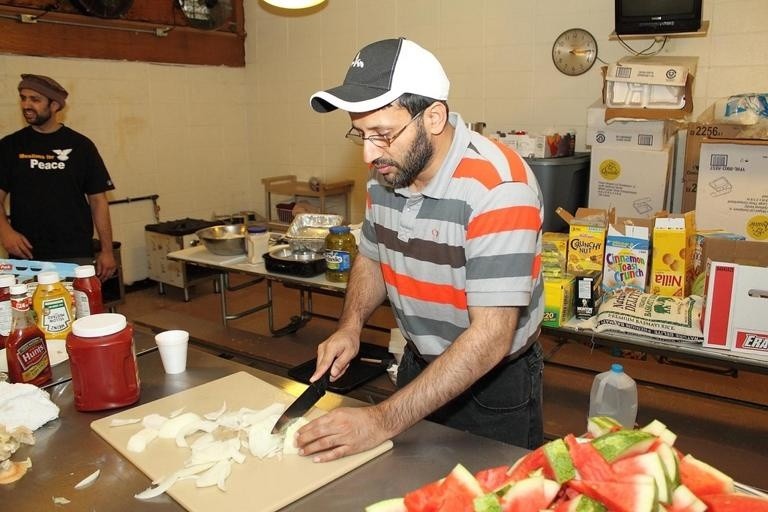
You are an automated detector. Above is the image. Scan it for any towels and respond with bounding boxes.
[0,380,59,432]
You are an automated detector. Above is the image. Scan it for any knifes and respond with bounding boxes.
[271,357,340,435]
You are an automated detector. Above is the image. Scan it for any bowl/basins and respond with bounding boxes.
[196,225,246,256]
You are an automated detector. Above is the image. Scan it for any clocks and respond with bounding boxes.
[552,28,598,77]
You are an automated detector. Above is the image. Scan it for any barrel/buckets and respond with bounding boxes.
[589,365,639,430]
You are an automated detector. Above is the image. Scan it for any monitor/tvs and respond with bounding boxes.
[614,0,703,35]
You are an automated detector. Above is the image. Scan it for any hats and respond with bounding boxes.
[18,73,68,109]
[309,38,450,113]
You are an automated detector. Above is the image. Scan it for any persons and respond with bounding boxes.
[0,73,118,284]
[291,35,546,465]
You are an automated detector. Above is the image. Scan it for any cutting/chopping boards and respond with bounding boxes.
[89,370,393,511]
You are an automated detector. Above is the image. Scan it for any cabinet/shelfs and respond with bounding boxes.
[1,0,246,68]
[261,174,355,226]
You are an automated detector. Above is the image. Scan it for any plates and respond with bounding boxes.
[286,213,342,252]
[263,245,327,278]
[288,341,395,394]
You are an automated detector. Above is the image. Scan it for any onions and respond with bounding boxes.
[109,418,141,428]
[74,469,101,489]
[126,402,309,500]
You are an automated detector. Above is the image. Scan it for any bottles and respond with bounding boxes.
[0,275,17,350]
[5,284,52,386]
[66,313,141,411]
[32,272,73,367]
[72,265,104,320]
[325,226,356,282]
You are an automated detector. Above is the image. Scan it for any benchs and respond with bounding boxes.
[360,374,582,440]
[133,307,320,368]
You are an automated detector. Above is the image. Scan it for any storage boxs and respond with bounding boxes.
[585,95,674,151]
[525,153,590,231]
[681,123,753,213]
[694,143,768,243]
[588,144,671,219]
[499,133,576,158]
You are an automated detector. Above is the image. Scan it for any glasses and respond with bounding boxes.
[345,111,424,147]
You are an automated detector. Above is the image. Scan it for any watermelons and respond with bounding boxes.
[567,473,659,512]
[476,465,511,496]
[583,415,622,436]
[402,475,446,512]
[699,492,768,512]
[554,494,605,512]
[613,451,672,505]
[654,441,680,487]
[664,484,708,512]
[589,429,660,464]
[676,451,735,494]
[499,477,561,512]
[641,419,676,446]
[511,439,577,483]
[422,463,483,512]
[472,491,503,511]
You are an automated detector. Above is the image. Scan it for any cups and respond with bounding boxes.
[155,330,190,375]
